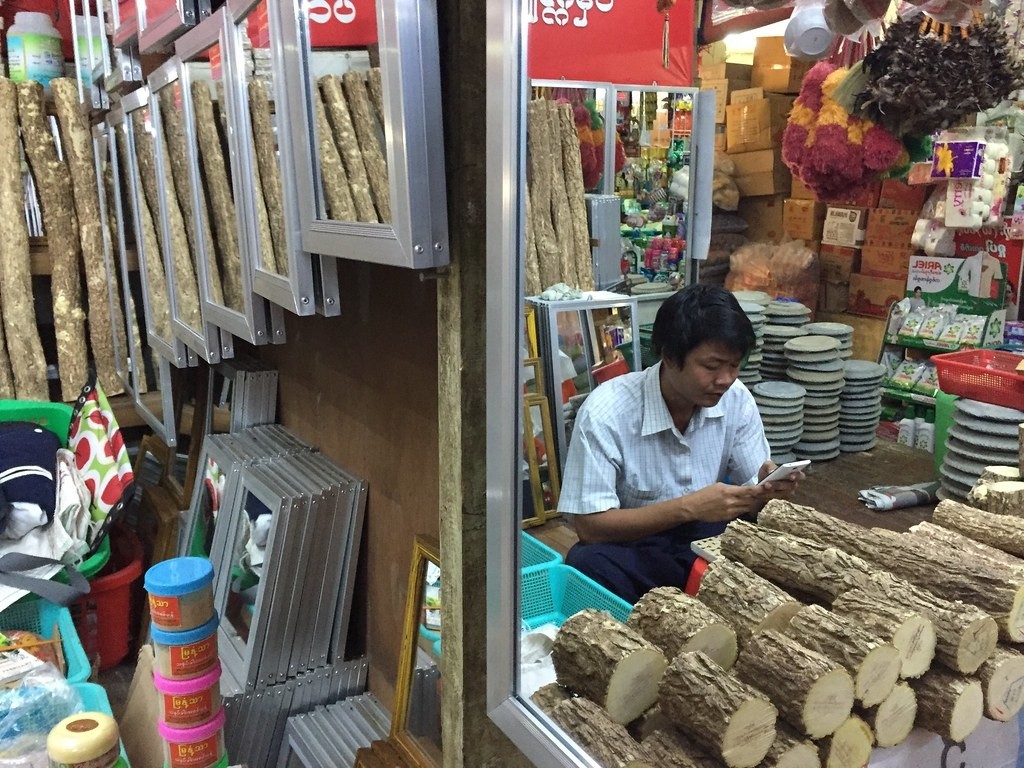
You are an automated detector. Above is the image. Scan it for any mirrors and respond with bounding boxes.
[89,0,443,768]
[521,304,561,528]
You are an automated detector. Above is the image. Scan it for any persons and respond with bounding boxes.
[1004,280,1018,321]
[556,283,807,606]
[909,287,926,313]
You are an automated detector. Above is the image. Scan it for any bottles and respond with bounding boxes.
[9,11,65,104]
[619,226,686,291]
[885,399,936,454]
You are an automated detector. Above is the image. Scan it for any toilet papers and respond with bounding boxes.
[910,136,1014,257]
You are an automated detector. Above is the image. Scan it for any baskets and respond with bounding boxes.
[930,348,1023,412]
[0,598,130,768]
[420,529,633,660]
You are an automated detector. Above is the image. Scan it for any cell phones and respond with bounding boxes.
[756,459,812,486]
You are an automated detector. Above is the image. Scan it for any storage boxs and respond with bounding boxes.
[632,34,932,364]
[0,398,143,768]
[930,348,1024,411]
[419,530,641,658]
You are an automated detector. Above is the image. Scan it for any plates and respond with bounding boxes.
[784,9,837,61]
[731,291,888,466]
[621,273,673,294]
[936,396,1024,503]
